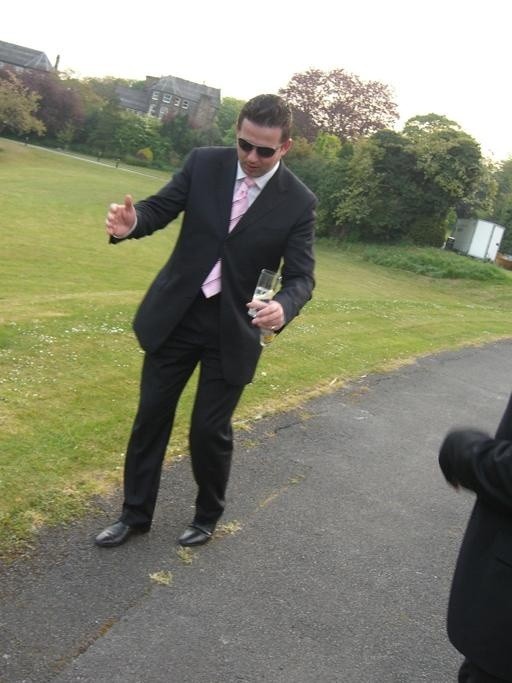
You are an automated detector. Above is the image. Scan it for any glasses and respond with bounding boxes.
[237,137,285,158]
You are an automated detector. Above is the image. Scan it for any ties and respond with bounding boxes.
[200,175,257,299]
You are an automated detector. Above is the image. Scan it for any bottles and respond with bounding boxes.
[257,294,275,348]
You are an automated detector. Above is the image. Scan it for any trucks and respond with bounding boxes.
[444,216,506,263]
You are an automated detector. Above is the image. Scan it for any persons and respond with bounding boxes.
[93,94,317,548]
[438,393,512,683]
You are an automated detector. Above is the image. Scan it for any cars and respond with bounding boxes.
[493,251,511,270]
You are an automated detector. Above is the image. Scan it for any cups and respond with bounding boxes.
[249,268,281,321]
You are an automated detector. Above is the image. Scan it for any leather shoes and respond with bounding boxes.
[178,514,216,546]
[95,518,151,548]
